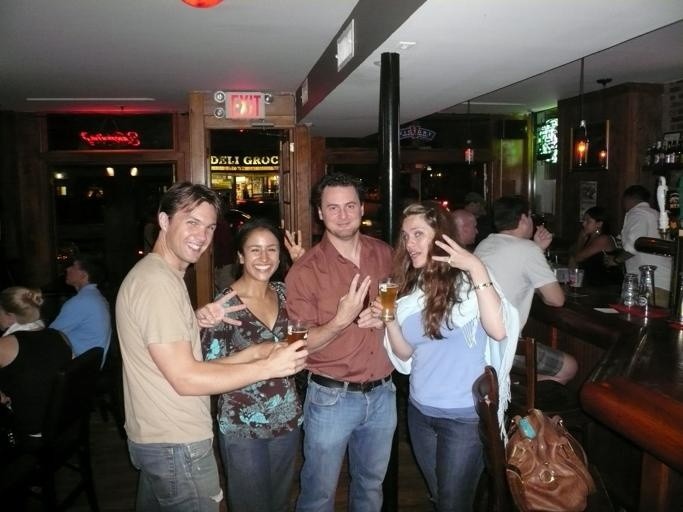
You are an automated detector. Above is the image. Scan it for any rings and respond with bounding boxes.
[369,302,375,307]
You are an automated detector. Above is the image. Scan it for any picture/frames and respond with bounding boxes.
[569,120,609,173]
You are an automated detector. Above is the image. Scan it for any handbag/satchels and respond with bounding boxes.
[1,403,24,450]
[506,408,595,511]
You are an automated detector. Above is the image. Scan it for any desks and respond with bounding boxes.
[580,369,682,511]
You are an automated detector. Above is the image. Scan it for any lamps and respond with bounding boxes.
[575,58,589,167]
[465,100,475,165]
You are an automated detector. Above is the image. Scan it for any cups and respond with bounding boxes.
[569,265,585,295]
[287,319,310,352]
[378,277,398,321]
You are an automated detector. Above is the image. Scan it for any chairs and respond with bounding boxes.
[472,366,616,511]
[0,347,105,512]
[505,336,593,456]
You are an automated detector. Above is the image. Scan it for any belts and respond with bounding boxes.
[312,374,390,392]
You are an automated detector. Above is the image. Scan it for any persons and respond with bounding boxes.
[369,202,520,511]
[575,188,672,308]
[112,179,311,510]
[47,243,114,378]
[197,221,308,512]
[454,192,579,385]
[284,169,406,510]
[0,286,73,455]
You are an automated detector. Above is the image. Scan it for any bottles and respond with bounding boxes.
[645,139,682,165]
[620,273,640,308]
[546,249,560,282]
[639,264,658,312]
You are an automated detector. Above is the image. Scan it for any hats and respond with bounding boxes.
[465,191,488,205]
